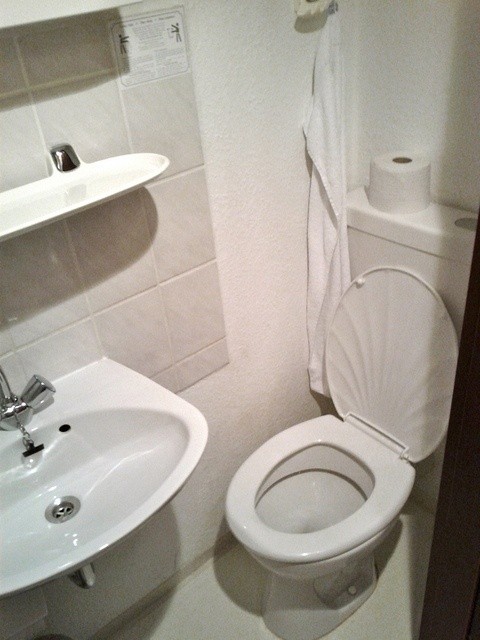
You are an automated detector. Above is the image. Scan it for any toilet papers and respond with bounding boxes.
[367,151,432,215]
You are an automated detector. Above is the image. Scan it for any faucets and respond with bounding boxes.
[0,364,57,430]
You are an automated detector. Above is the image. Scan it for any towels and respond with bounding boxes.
[300,11,350,398]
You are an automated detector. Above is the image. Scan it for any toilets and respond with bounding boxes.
[223,185,479,639]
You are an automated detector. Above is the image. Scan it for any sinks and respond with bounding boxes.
[0,357,209,596]
